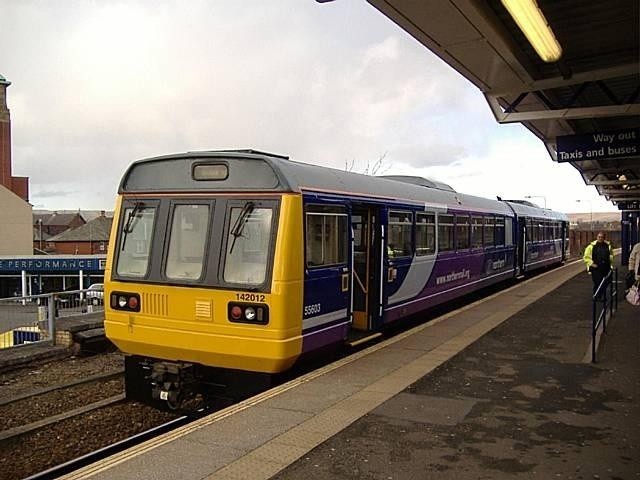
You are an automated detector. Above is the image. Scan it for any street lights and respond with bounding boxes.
[525,196,546,209]
[576,200,592,221]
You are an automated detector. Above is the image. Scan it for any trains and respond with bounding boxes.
[103,149,571,416]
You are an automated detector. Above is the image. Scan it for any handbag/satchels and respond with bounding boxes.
[625,281,640,306]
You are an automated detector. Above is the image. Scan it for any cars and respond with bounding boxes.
[53,293,84,308]
[86,283,104,306]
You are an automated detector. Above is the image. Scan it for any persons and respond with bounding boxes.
[583,231,615,302]
[14,287,19,296]
[628,242,640,301]
[47,294,61,320]
